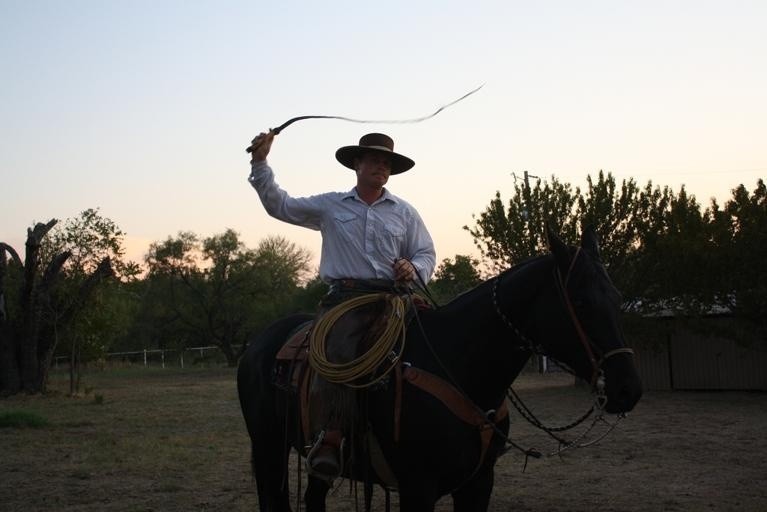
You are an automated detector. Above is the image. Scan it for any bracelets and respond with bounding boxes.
[255,160,268,170]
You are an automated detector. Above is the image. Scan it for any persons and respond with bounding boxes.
[248,129,436,476]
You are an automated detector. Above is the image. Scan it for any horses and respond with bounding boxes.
[236,219,644,512]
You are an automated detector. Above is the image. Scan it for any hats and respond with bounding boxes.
[335,134,415,175]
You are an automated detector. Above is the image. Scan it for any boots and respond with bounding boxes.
[310,387,342,475]
